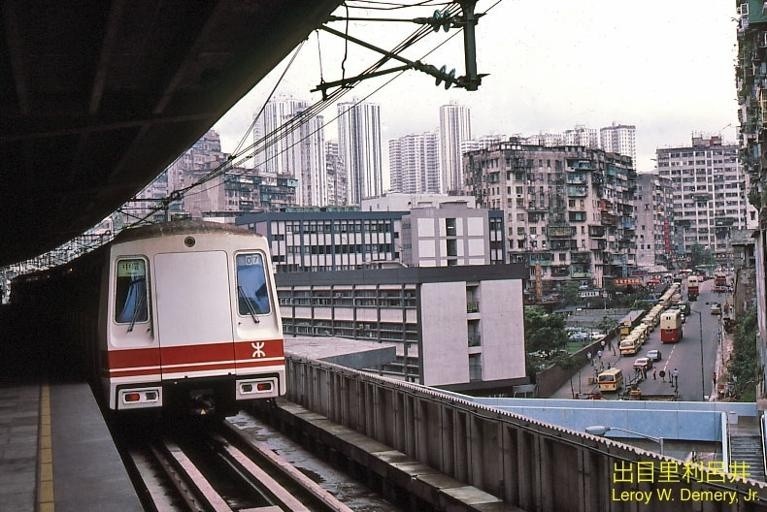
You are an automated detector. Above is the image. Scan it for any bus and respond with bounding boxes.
[596,273,699,394]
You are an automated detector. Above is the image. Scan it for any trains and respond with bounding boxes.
[7,220,288,437]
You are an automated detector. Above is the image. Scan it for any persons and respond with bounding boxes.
[635,365,648,379]
[729,303,733,313]
[672,368,680,378]
[652,366,657,380]
[585,339,611,375]
[712,371,717,386]
